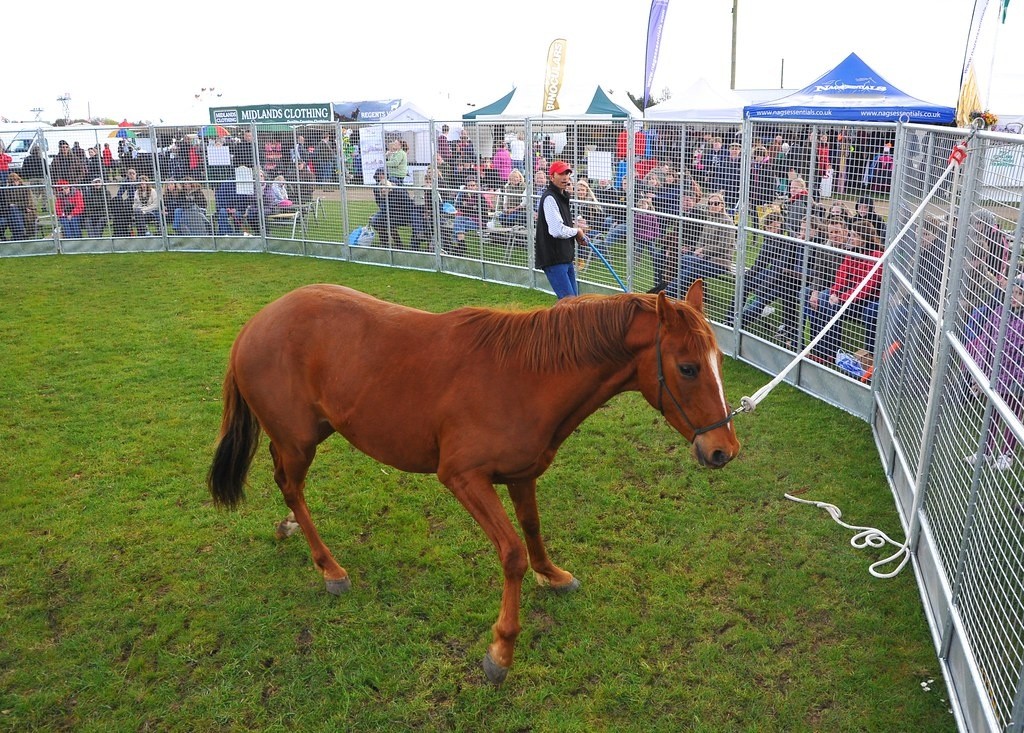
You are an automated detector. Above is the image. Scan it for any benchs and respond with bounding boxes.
[714,264,751,283]
[311,196,328,221]
[507,226,614,274]
[34,218,58,239]
[441,224,519,262]
[37,215,54,219]
[206,211,309,239]
[270,202,320,229]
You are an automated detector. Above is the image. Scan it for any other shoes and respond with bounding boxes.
[454,240,467,257]
[995,454,1014,470]
[321,186,334,192]
[963,452,993,465]
[136,229,152,237]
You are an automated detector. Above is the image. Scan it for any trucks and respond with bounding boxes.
[3,125,137,179]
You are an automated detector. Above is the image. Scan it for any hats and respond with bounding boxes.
[1012,273,1024,285]
[59,140,69,147]
[728,142,741,147]
[90,177,101,183]
[549,161,573,175]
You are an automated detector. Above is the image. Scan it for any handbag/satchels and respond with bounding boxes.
[349,221,375,247]
[835,349,865,377]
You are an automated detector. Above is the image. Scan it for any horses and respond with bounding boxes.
[204,278,744,687]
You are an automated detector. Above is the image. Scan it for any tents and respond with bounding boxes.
[462,53,954,175]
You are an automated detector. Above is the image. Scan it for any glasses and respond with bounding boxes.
[708,200,724,206]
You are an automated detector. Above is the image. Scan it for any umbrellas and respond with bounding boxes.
[108,129,138,141]
[197,126,230,136]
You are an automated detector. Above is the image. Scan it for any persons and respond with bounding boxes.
[370,125,901,367]
[0,117,336,241]
[725,177,1024,470]
